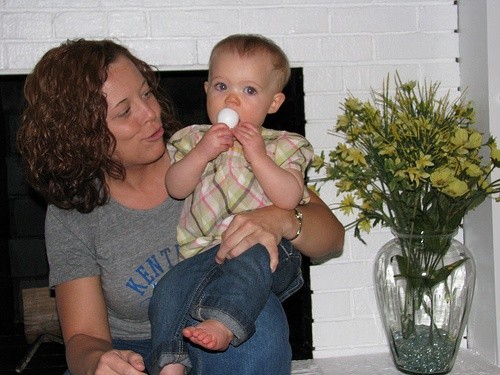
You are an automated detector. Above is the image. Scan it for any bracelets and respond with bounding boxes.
[287,205,305,241]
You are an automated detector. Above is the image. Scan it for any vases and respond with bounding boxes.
[372,228,476,375]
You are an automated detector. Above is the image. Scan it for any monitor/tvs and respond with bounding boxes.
[1,66,314,370]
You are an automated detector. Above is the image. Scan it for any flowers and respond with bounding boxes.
[301,70,499,340]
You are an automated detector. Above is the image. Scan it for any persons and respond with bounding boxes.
[150,34,315,375]
[17,39,344,375]
